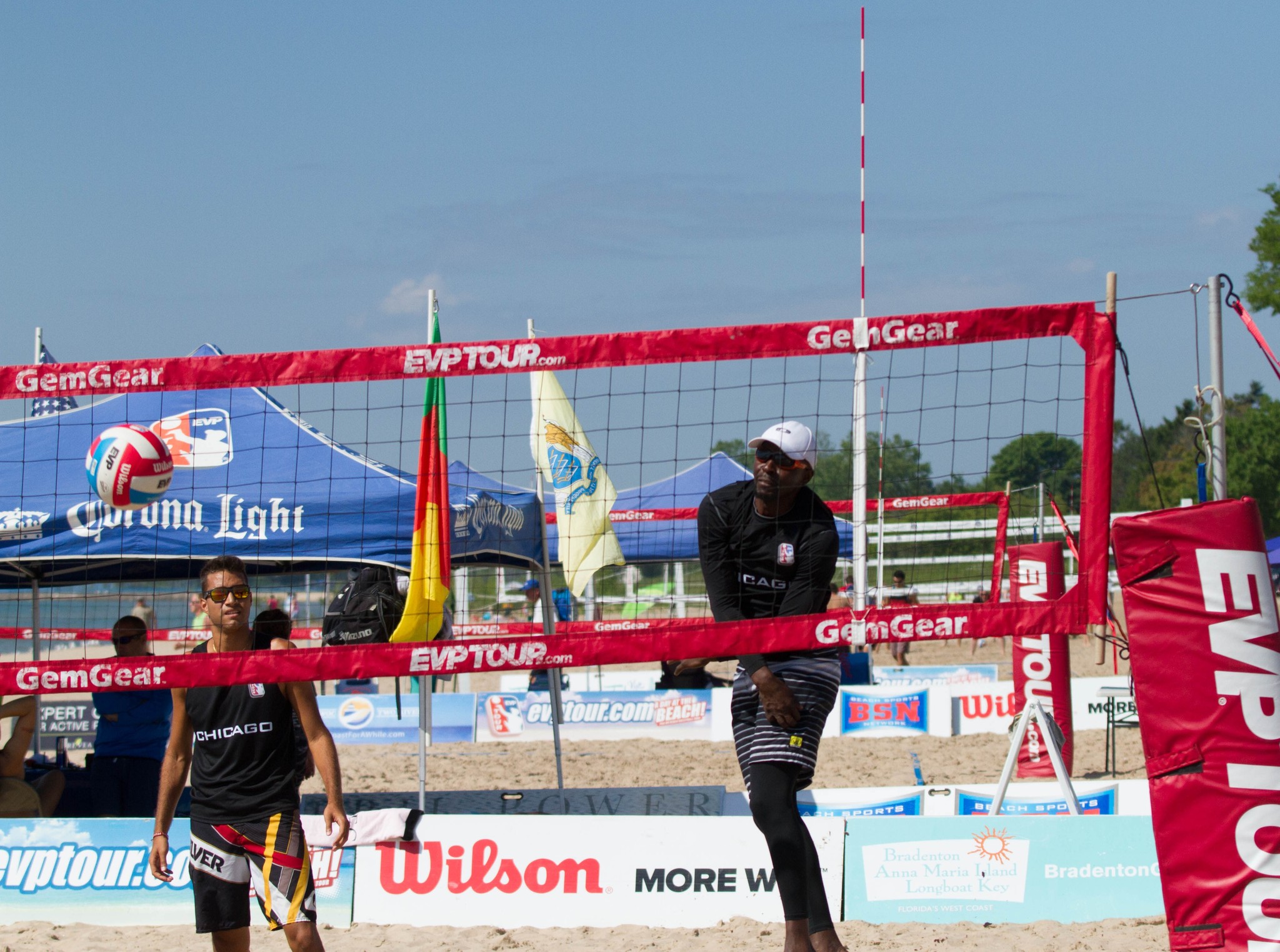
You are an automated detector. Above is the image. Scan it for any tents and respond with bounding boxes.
[1264,537,1280,566]
[0,340,734,816]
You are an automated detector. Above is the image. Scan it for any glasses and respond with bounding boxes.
[203,584,251,602]
[111,634,140,645]
[755,446,803,470]
[894,580,902,583]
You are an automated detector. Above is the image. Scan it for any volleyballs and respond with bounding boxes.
[84,423,174,512]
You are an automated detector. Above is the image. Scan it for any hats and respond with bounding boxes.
[520,579,540,590]
[748,421,818,471]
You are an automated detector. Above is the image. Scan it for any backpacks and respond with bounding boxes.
[318,562,405,645]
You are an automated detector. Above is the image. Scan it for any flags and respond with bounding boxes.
[526,319,625,598]
[384,282,452,645]
[31,329,77,415]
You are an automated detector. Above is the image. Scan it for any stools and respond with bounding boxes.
[1096,687,1139,777]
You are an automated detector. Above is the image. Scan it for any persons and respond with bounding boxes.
[0,555,350,952]
[667,420,846,952]
[518,579,570,692]
[826,570,1091,667]
